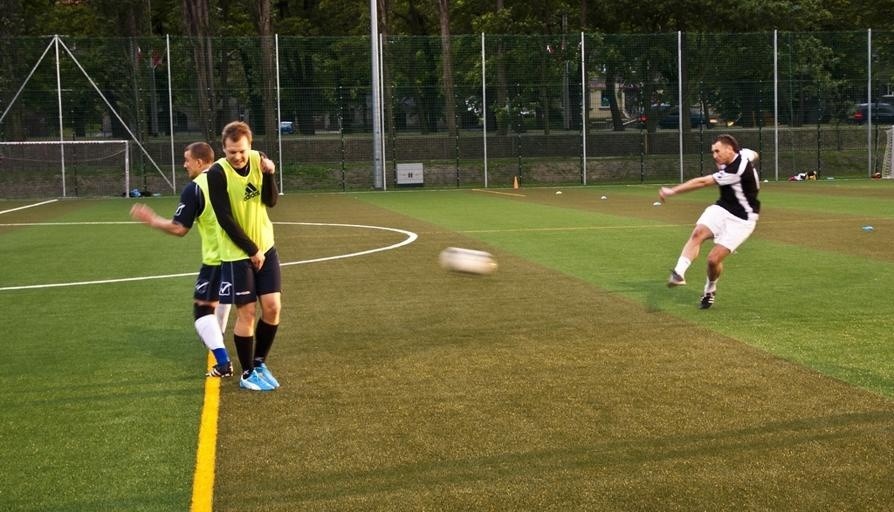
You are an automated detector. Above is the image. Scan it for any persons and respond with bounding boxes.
[129,142,234,378]
[659,134,761,309]
[207,121,283,391]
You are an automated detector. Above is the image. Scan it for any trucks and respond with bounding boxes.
[846,92,894,126]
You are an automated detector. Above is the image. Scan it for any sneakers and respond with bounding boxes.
[667,273,687,288]
[699,292,716,308]
[240,362,280,391]
[205,362,234,378]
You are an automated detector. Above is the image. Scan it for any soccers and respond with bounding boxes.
[437,246,498,277]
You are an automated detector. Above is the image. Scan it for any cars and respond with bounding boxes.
[634,102,707,130]
